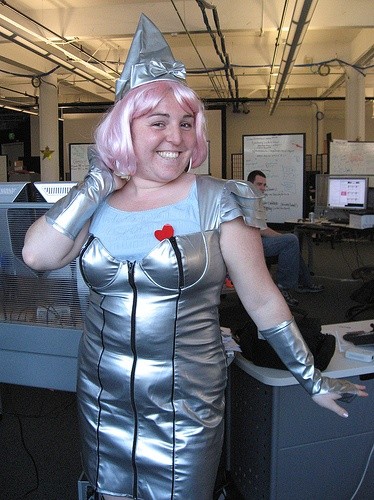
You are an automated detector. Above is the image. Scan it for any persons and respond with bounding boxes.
[22,12,368,500]
[247,170,325,307]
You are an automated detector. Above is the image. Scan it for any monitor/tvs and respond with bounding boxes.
[326,176,369,224]
[314,174,327,214]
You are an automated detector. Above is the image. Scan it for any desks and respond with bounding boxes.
[271,219,374,276]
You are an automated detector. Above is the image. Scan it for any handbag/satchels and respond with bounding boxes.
[230,301,336,371]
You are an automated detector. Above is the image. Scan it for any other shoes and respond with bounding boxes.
[294,280,326,293]
[277,284,299,307]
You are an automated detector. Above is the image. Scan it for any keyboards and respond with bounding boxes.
[297,223,340,232]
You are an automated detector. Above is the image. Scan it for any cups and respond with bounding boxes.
[309,212,314,223]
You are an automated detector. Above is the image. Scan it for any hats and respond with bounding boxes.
[114,12,189,104]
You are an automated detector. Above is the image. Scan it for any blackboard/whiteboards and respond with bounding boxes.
[241,131,307,230]
[326,138,374,188]
[189,139,211,175]
[68,142,98,182]
